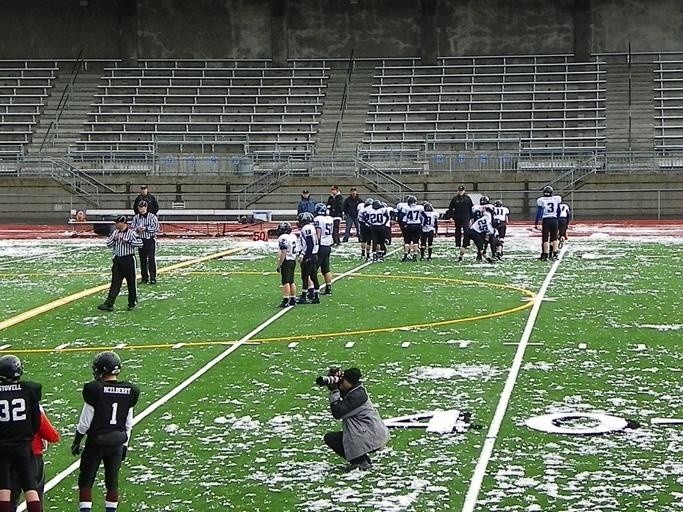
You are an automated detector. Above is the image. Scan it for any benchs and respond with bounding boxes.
[591,51,681,62]
[287,58,423,67]
[66,203,480,238]
[1,68,58,177]
[355,62,607,172]
[69,66,332,174]
[653,61,682,172]
[138,59,273,68]
[436,54,575,66]
[1,59,122,69]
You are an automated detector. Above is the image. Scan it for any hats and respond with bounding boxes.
[457,186,465,191]
[330,186,339,190]
[112,215,127,223]
[344,368,361,385]
[302,190,309,194]
[137,200,147,207]
[350,187,357,192]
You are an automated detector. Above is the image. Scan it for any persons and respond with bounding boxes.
[130,200,160,285]
[559,203,570,248]
[277,184,511,308]
[535,186,562,260]
[324,366,390,470]
[0,354,42,512]
[70,351,140,512]
[98,216,144,312]
[30,406,60,512]
[133,184,159,218]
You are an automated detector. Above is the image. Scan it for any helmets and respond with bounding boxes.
[314,203,326,215]
[364,195,433,211]
[479,197,502,228]
[92,351,121,379]
[296,212,315,228]
[543,186,555,197]
[0,354,23,382]
[276,222,291,236]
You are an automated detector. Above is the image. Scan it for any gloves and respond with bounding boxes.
[121,446,127,461]
[71,442,82,457]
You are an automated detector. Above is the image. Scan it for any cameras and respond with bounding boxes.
[316,366,343,391]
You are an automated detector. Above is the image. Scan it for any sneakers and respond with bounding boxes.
[150,280,155,284]
[97,304,113,311]
[127,305,135,310]
[558,236,564,250]
[276,290,334,308]
[345,456,373,472]
[139,279,148,284]
[359,254,560,264]
